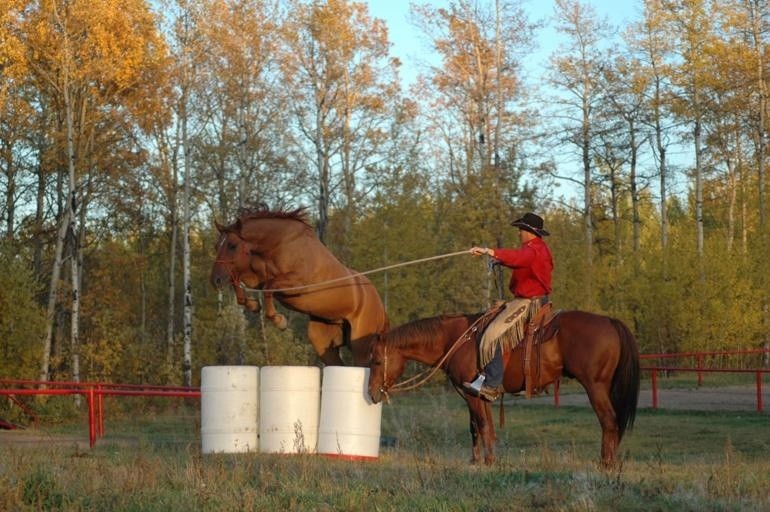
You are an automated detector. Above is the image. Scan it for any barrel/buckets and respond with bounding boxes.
[260,366,320,458]
[200,365,260,453]
[318,365,384,461]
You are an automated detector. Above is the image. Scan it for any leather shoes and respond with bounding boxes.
[462,382,500,402]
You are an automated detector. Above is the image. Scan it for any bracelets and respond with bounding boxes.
[483,248,488,255]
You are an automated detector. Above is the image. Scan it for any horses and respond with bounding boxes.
[210,205,390,367]
[367,310,641,468]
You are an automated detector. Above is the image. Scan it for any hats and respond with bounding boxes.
[510,212,550,236]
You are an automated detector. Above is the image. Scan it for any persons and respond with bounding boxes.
[463,212,553,402]
